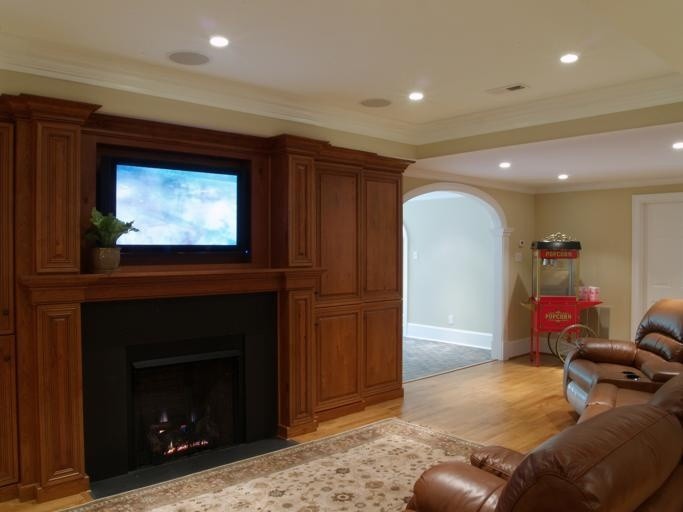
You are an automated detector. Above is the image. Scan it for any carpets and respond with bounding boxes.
[52,417,484,512]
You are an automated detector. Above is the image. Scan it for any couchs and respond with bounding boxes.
[404,298,683,512]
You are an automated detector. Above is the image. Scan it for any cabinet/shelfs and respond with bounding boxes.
[0,88,414,507]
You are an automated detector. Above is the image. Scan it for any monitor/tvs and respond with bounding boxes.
[99,156,249,252]
